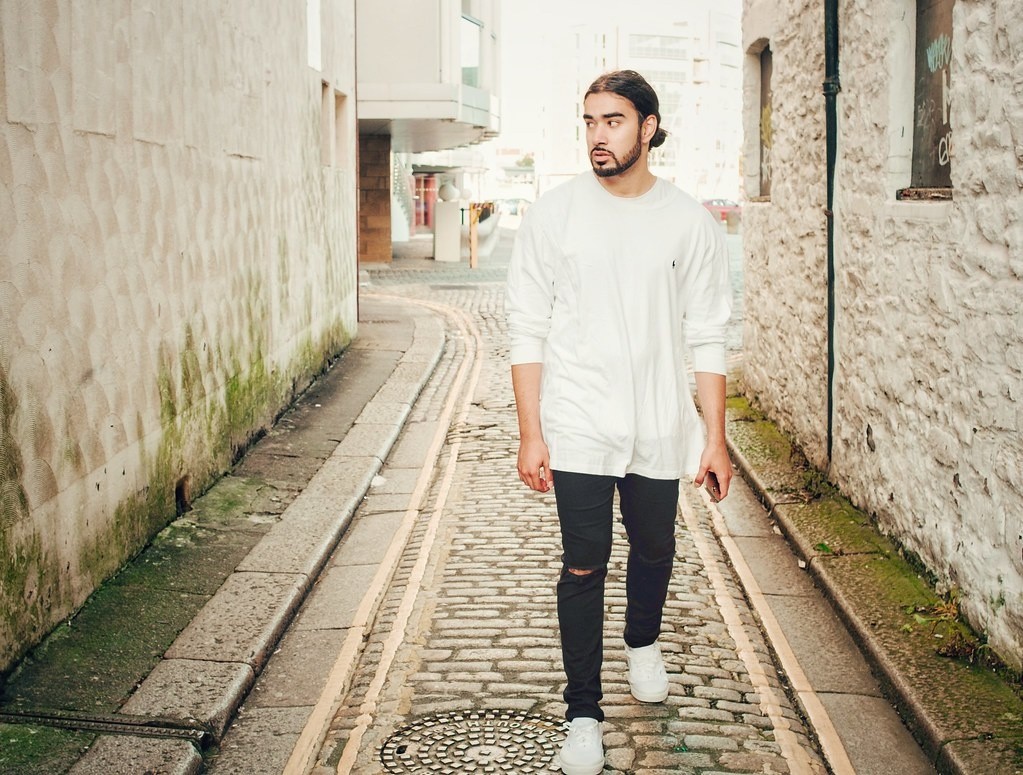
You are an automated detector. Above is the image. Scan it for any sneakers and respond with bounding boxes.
[557,717,606,775]
[624,637,670,702]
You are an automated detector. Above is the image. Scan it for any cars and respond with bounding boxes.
[702,199,742,221]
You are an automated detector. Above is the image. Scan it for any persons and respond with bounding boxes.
[504,69,733,775]
